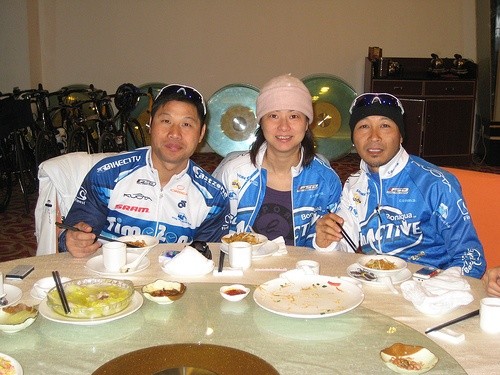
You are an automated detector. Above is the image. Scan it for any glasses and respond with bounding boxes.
[349,93,404,115]
[155,85,207,115]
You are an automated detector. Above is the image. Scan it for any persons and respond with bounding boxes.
[57,83,230,257]
[211,76,343,249]
[312,93,489,280]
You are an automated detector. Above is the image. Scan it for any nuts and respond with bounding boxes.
[390,357,421,371]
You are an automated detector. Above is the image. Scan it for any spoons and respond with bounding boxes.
[120,247,150,273]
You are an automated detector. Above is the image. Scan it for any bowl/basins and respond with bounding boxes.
[0,315,38,334]
[117,234,159,256]
[35,276,71,298]
[358,255,408,277]
[221,233,268,251]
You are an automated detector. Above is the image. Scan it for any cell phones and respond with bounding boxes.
[413,267,441,280]
[5,264,35,280]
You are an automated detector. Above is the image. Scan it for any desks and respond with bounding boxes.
[0,242,500,375]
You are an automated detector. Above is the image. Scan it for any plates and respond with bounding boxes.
[220,242,279,258]
[253,275,364,318]
[346,262,412,286]
[47,278,134,320]
[86,253,150,276]
[39,290,144,326]
[220,284,251,301]
[31,288,44,300]
[0,284,23,309]
[380,343,438,375]
[0,353,23,375]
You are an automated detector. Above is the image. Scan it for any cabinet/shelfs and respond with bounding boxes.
[364,56,480,164]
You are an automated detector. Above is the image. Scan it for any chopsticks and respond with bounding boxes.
[425,309,480,334]
[218,251,225,272]
[55,222,140,248]
[327,209,358,253]
[52,270,71,314]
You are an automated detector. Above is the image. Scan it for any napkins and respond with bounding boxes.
[271,236,287,257]
[403,264,472,319]
[160,245,214,277]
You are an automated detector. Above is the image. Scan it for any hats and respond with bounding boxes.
[256,73,314,124]
[349,101,406,144]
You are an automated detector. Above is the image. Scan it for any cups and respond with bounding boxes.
[102,242,127,273]
[296,260,319,274]
[228,242,252,271]
[479,297,500,335]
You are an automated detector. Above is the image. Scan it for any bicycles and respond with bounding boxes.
[1,83,153,211]
